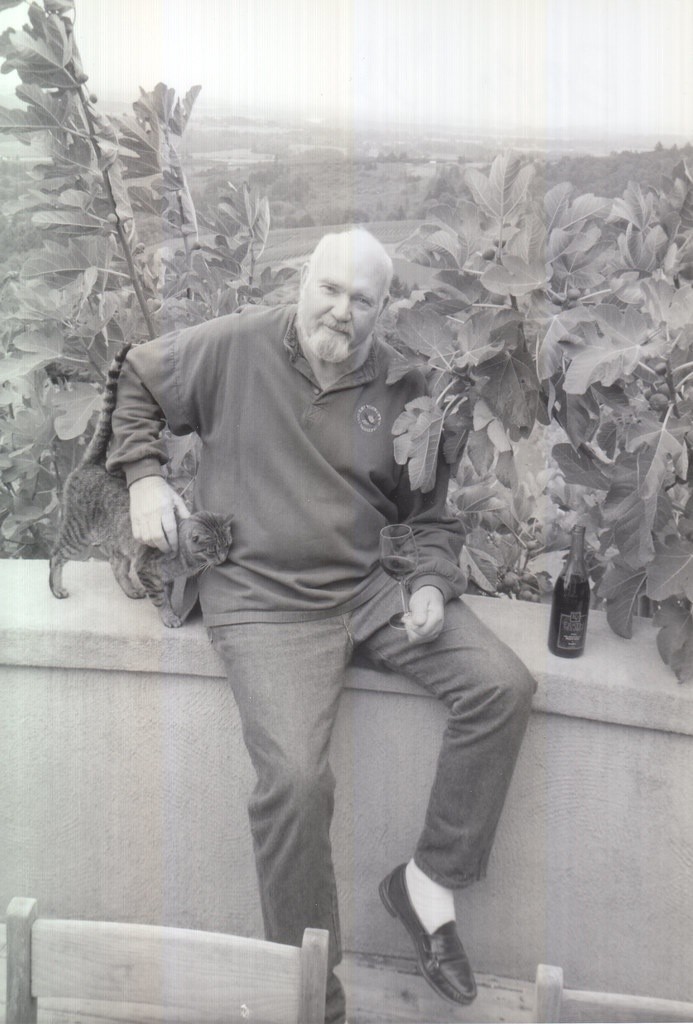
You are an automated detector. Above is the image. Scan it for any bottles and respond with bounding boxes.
[548,525,591,658]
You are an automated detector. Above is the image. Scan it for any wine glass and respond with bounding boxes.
[379,524,419,629]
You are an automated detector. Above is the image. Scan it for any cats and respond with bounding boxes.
[49,341,236,628]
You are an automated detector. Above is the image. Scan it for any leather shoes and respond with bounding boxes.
[378,863,477,1006]
[323,988,347,1024]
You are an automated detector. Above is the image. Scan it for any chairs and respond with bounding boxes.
[3,895,692,1023]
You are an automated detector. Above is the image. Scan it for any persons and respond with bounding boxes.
[101,224,538,1024]
